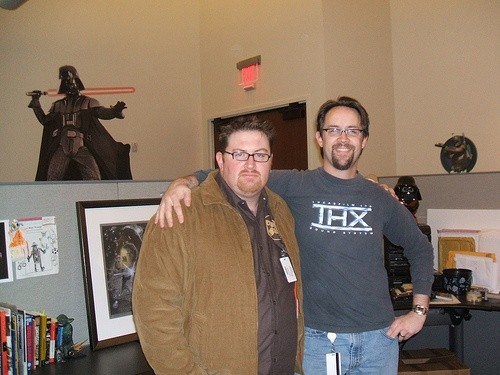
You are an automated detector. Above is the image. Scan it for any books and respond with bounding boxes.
[0,302,63,375]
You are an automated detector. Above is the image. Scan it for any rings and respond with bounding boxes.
[399,333,406,338]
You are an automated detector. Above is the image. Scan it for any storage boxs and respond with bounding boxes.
[399,348,471,375]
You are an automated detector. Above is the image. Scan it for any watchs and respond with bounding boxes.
[411,304,428,315]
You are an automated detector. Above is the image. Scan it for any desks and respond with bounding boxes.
[391,292,500,375]
[24,341,152,375]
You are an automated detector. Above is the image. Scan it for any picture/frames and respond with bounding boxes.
[75,198,166,351]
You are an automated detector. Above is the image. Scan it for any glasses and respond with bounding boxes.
[222,150,272,162]
[320,128,365,137]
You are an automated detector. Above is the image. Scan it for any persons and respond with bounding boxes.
[27,66,132,181]
[155,96,434,375]
[131,117,307,375]
